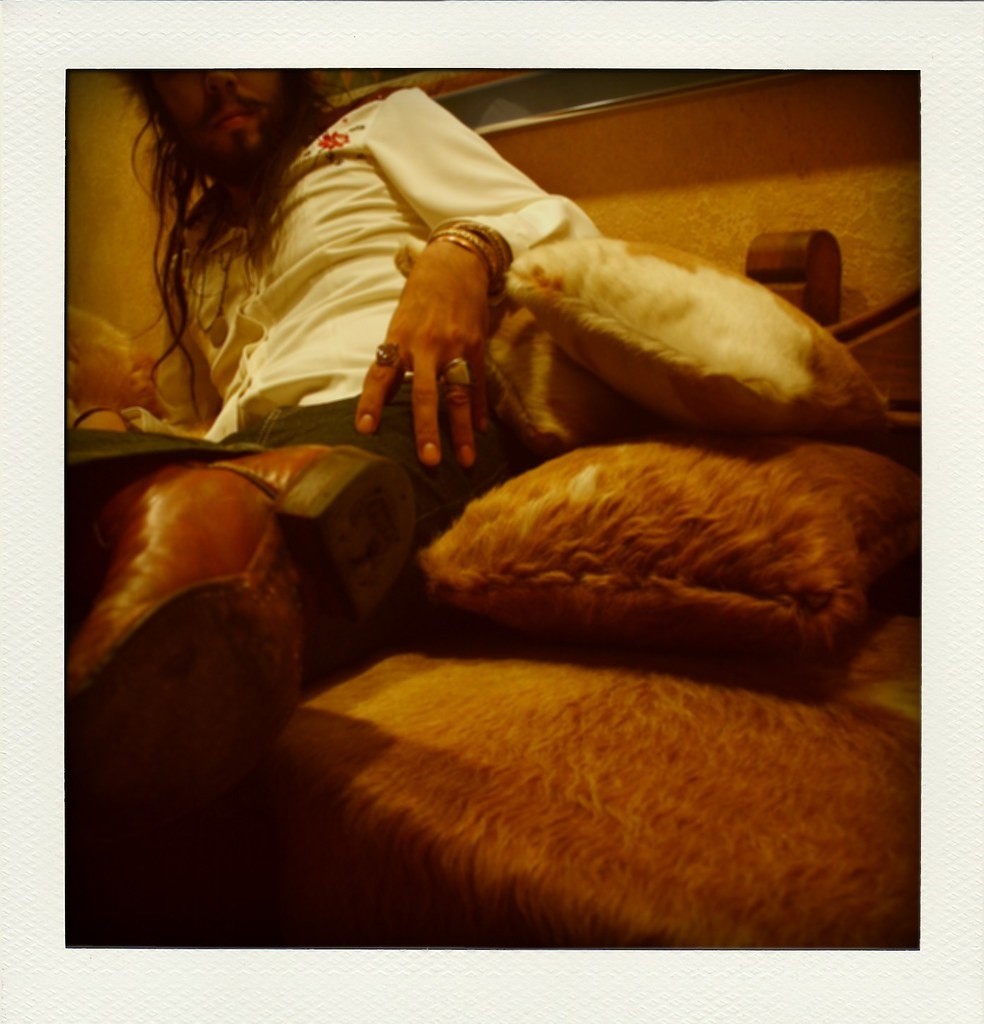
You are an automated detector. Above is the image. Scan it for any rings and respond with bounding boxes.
[374,343,401,365]
[444,358,470,384]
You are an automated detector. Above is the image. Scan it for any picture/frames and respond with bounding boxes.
[311,68,791,136]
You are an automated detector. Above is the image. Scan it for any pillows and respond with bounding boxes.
[397,246,665,458]
[418,431,921,680]
[501,235,889,444]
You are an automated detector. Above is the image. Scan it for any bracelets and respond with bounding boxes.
[427,220,512,292]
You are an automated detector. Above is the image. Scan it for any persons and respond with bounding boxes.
[110,67,600,648]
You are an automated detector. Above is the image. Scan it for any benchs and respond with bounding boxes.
[232,222,919,949]
